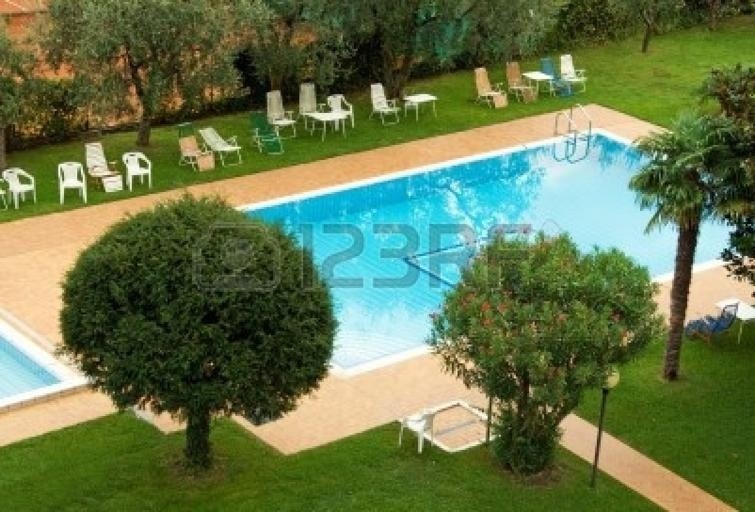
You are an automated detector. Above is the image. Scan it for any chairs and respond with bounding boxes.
[0,52,588,210]
[398,408,437,453]
[715,297,755,344]
[685,302,740,345]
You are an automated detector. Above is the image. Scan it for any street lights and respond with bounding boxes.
[589,365,623,487]
